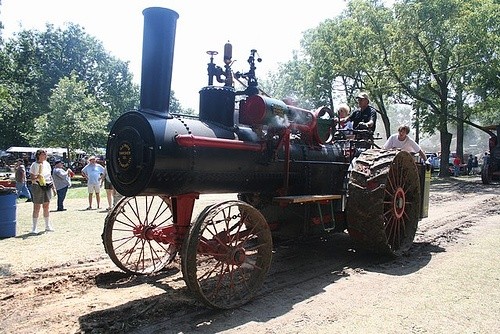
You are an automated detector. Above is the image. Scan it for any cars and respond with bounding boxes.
[412,151,469,173]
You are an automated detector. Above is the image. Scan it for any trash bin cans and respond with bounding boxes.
[0,187,17,238]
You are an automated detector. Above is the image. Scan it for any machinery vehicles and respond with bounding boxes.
[102,6,430,309]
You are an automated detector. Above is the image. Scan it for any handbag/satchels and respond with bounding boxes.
[46,182,53,190]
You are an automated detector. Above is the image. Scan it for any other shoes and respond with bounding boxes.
[29,230,38,235]
[57,208,67,211]
[46,227,54,232]
[26,198,32,202]
[86,207,113,210]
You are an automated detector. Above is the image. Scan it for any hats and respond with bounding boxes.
[15,159,25,164]
[87,156,97,162]
[356,92,369,100]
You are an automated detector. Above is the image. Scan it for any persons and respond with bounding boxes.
[331,92,377,158]
[454,155,460,176]
[101,164,116,211]
[15,158,32,202]
[334,107,353,139]
[483,151,491,164]
[29,149,57,235]
[81,156,106,210]
[53,160,72,211]
[467,154,473,175]
[380,125,432,165]
[473,156,478,168]
